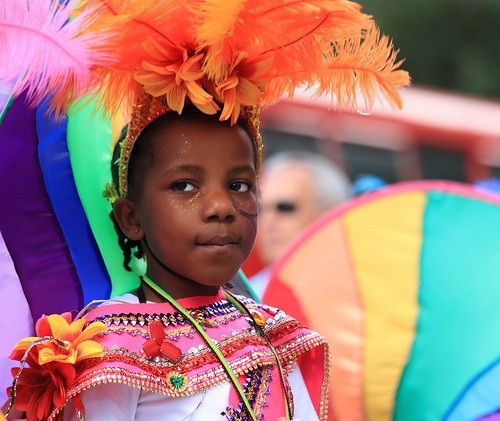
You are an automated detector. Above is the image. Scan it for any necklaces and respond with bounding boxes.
[136,273,294,420]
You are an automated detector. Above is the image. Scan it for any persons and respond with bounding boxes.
[2,101,332,421]
[245,150,352,300]
